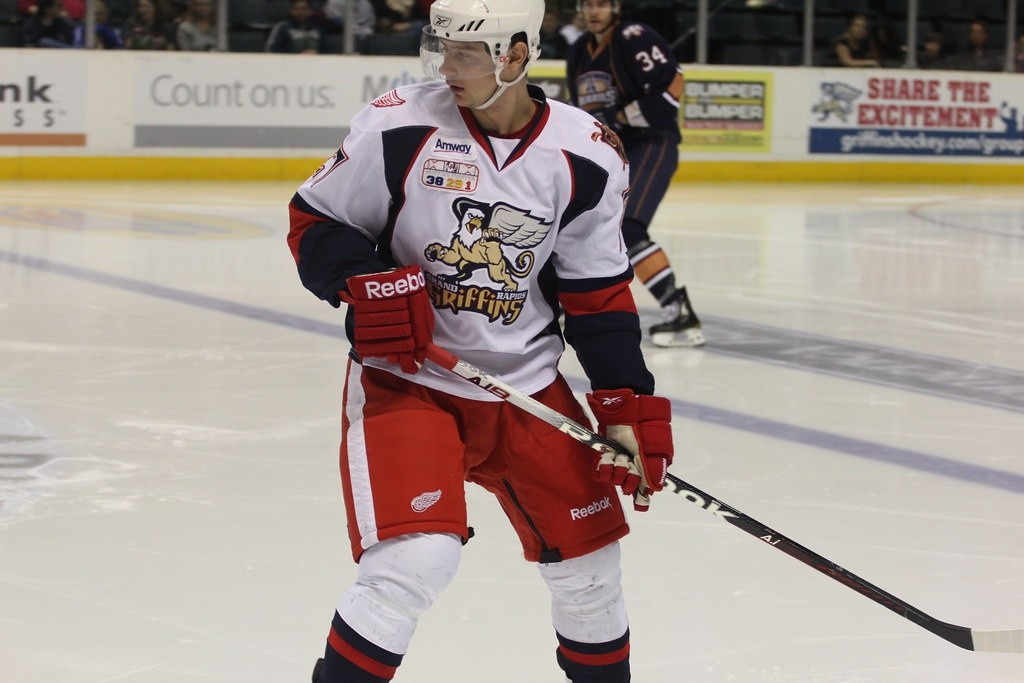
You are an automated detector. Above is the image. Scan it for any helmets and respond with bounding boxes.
[576,0,621,15]
[419,0,545,87]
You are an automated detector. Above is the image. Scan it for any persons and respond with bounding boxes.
[284,0,673,683]
[1,0,1024,74]
[564,0,709,349]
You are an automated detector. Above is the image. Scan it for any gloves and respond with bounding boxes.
[585,388,674,513]
[345,264,435,375]
[588,104,628,136]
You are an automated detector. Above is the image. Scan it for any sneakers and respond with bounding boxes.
[646,286,707,348]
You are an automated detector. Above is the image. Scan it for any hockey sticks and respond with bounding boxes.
[422,342,1024,655]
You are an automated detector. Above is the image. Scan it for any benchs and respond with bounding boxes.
[629,0,1024,74]
[225,4,427,58]
